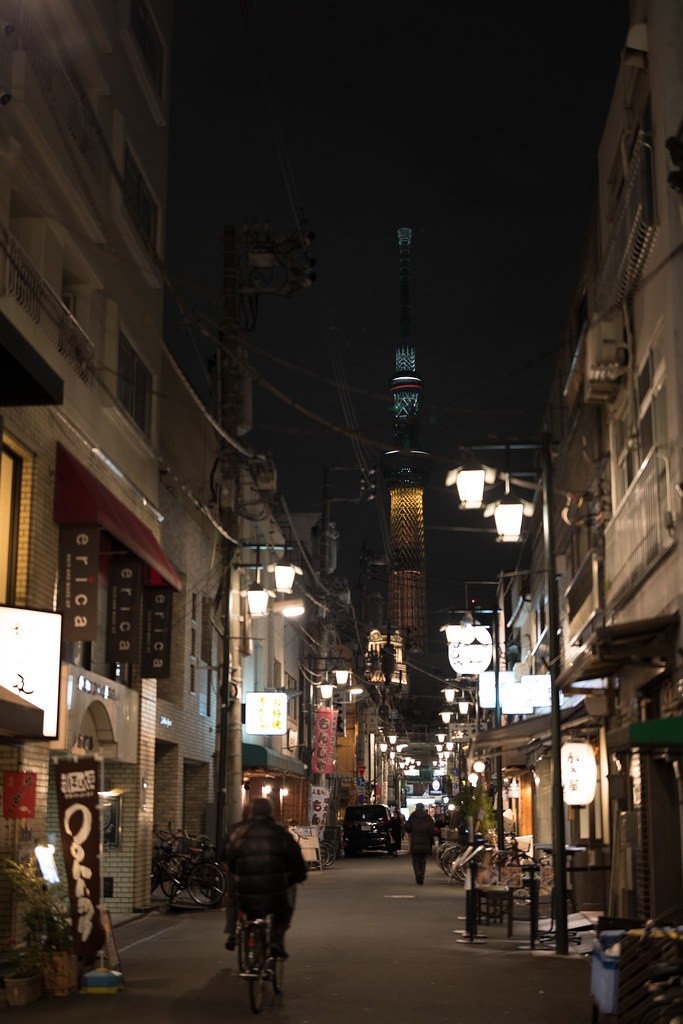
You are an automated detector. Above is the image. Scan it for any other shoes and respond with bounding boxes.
[417,873,424,885]
[225,935,236,951]
[268,944,290,961]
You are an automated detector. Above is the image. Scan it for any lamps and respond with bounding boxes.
[316,680,337,699]
[332,660,353,684]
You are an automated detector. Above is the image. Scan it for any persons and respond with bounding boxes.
[220,799,309,959]
[382,802,449,885]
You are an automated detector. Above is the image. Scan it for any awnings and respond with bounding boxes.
[53,441,182,589]
[0,310,63,407]
[469,612,683,754]
[240,742,305,778]
[0,686,44,739]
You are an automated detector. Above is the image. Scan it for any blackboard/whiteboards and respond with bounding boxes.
[100,909,121,969]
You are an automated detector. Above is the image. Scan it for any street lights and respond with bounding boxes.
[445,424,569,955]
[373,728,404,803]
[437,677,481,790]
[297,640,364,762]
[439,597,507,865]
[213,508,307,868]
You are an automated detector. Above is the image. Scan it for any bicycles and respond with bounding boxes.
[435,824,586,917]
[232,909,285,1014]
[286,818,337,868]
[151,820,226,906]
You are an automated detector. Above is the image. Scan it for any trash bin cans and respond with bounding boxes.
[590,930,628,1024]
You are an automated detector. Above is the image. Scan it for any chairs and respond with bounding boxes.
[470,859,513,938]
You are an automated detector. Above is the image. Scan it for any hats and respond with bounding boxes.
[434,813,441,818]
[253,798,271,814]
[392,810,400,814]
[415,803,424,808]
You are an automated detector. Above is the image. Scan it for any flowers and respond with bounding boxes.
[5,937,23,977]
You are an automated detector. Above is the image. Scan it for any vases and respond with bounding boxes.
[4,970,42,1005]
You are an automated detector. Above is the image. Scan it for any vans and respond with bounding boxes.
[342,804,395,857]
